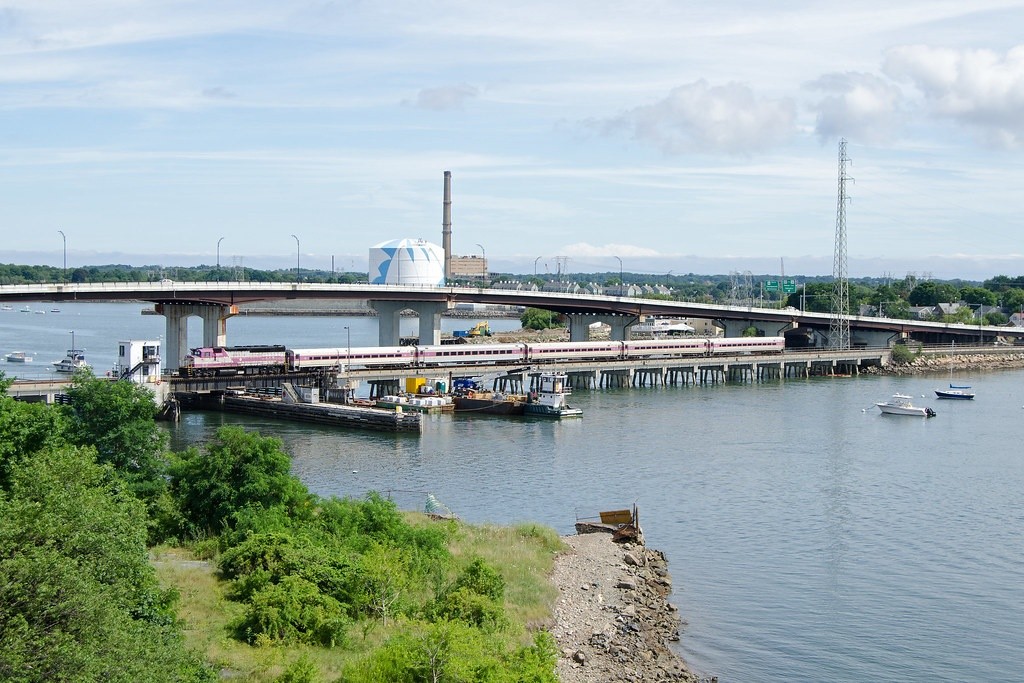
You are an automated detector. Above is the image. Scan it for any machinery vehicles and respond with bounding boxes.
[467,320,492,338]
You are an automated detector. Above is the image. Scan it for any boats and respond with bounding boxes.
[448,363,585,418]
[574,502,646,546]
[934,390,976,400]
[3,305,61,314]
[369,375,454,413]
[52,331,91,372]
[876,392,929,416]
[6,351,33,362]
[949,384,972,390]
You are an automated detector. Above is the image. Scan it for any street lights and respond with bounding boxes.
[344,325,350,372]
[291,234,299,280]
[217,236,225,268]
[58,230,67,269]
[476,243,485,288]
[615,255,624,296]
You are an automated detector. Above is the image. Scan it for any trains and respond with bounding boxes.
[179,334,786,378]
